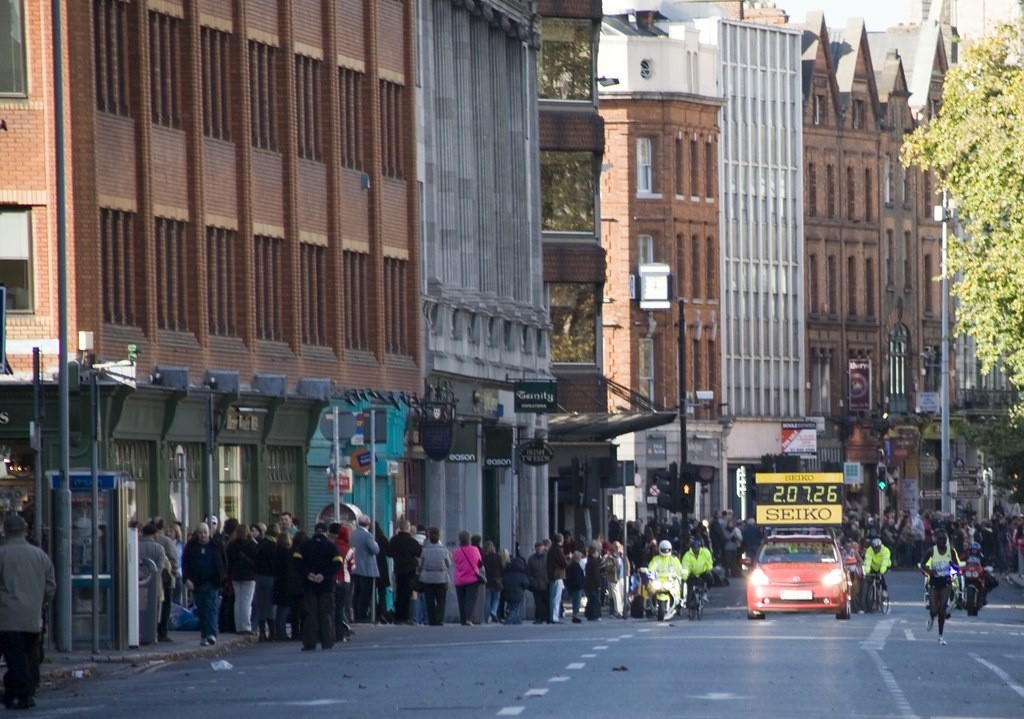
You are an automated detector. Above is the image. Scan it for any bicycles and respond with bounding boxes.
[864,571,890,615]
[685,572,711,621]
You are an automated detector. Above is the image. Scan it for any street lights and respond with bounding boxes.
[931,188,958,514]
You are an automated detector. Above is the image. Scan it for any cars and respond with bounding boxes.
[738,524,862,620]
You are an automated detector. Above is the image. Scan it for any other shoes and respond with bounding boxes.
[361,609,630,625]
[207,634,216,644]
[927,620,933,631]
[258,634,303,643]
[322,644,332,650]
[158,635,174,642]
[200,638,210,646]
[300,645,316,651]
[3,692,36,710]
[939,638,947,645]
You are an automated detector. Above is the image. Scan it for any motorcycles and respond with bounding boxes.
[917,560,994,616]
[638,561,688,622]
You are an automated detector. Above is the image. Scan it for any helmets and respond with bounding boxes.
[969,542,981,550]
[659,540,672,556]
[871,538,881,547]
[690,540,701,546]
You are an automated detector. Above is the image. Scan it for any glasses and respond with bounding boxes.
[212,522,217,524]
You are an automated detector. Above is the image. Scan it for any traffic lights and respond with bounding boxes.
[876,463,887,491]
[678,463,697,513]
[557,456,580,509]
[656,461,678,515]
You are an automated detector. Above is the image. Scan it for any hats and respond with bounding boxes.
[314,523,328,532]
[205,516,218,524]
[256,522,267,532]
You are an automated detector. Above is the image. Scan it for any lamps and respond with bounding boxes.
[596,76,620,87]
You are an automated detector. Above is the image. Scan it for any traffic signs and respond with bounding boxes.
[919,489,941,497]
[952,489,981,500]
[952,465,978,476]
[956,476,982,486]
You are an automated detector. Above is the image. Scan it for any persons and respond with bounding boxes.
[0,499,611,710]
[388,520,421,625]
[921,528,960,646]
[293,523,343,651]
[963,501,976,524]
[681,538,713,610]
[454,531,483,625]
[350,515,380,623]
[647,540,683,594]
[528,542,549,624]
[993,499,1004,515]
[548,534,573,624]
[227,524,255,636]
[332,526,356,643]
[606,541,631,616]
[182,522,225,646]
[416,532,451,627]
[606,499,1024,604]
[857,538,892,613]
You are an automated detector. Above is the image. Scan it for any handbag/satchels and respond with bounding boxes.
[409,574,423,592]
[493,577,504,591]
[477,574,487,584]
